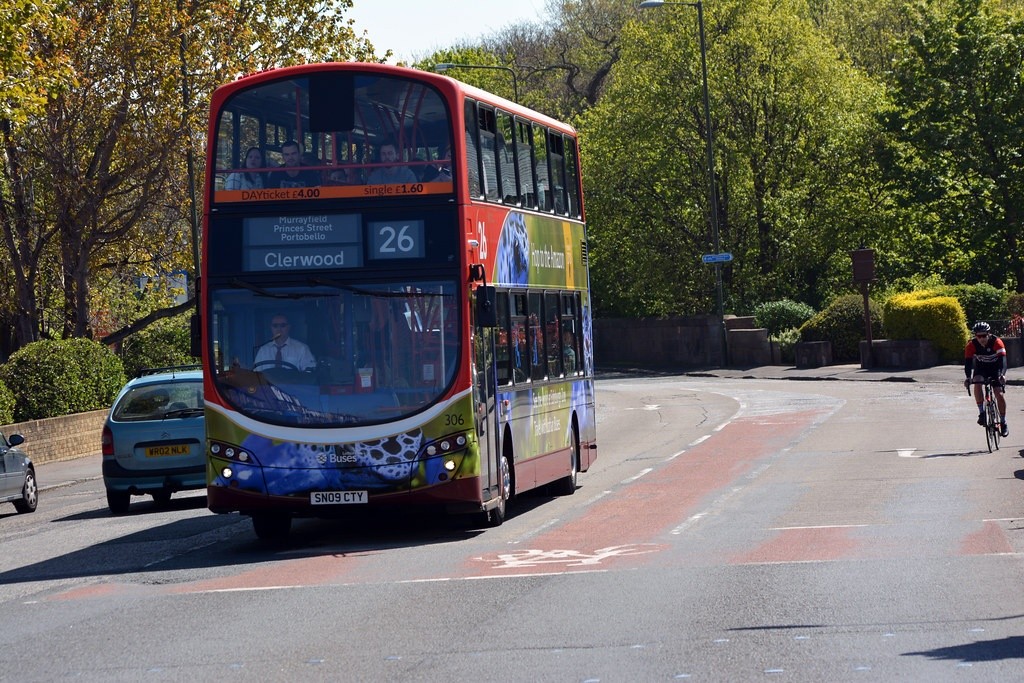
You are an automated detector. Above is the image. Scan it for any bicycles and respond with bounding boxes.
[965,374,1005,452]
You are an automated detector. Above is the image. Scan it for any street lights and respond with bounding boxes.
[434,63,518,104]
[639,0,730,368]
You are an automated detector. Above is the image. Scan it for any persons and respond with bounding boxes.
[962,321,1011,439]
[366,141,419,186]
[223,147,266,190]
[421,145,452,183]
[251,313,316,378]
[297,149,425,186]
[266,139,321,191]
[562,329,576,376]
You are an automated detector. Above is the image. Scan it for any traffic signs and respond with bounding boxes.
[702,253,733,263]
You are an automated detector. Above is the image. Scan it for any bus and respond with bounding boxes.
[190,63,597,545]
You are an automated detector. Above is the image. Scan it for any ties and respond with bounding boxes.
[272,344,286,369]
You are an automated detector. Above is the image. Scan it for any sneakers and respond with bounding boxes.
[978,412,984,425]
[1001,422,1008,437]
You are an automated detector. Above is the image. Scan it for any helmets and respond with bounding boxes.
[972,322,991,334]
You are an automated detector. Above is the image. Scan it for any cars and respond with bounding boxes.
[0,430,38,513]
[101,364,208,515]
